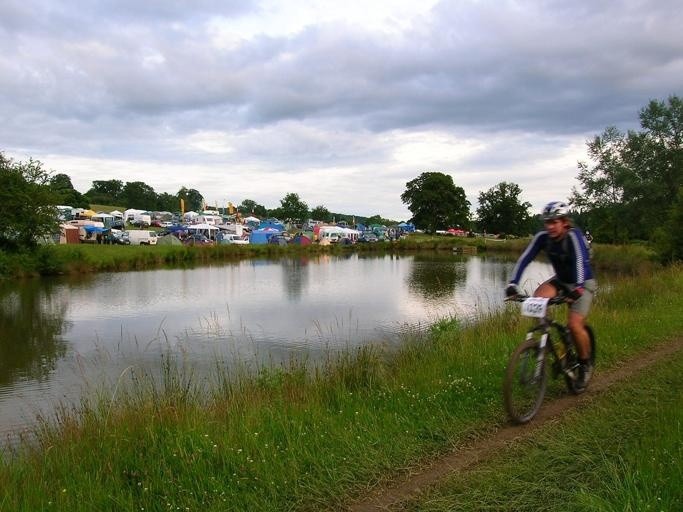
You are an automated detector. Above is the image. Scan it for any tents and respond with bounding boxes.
[258,222,287,232]
[249,226,279,244]
[243,220,260,230]
[212,230,237,245]
[286,235,312,245]
[352,221,415,242]
[166,223,191,237]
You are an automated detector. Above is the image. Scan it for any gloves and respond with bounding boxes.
[565,289,584,301]
[506,286,518,296]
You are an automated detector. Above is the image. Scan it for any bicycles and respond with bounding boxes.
[502,292,597,423]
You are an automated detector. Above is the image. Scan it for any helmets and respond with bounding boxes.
[541,201,570,221]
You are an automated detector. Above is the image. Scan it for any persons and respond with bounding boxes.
[395,248,399,260]
[312,223,319,241]
[389,248,394,260]
[503,199,597,391]
[78,232,116,247]
[582,230,593,248]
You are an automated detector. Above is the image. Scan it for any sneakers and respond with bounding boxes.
[576,364,592,388]
